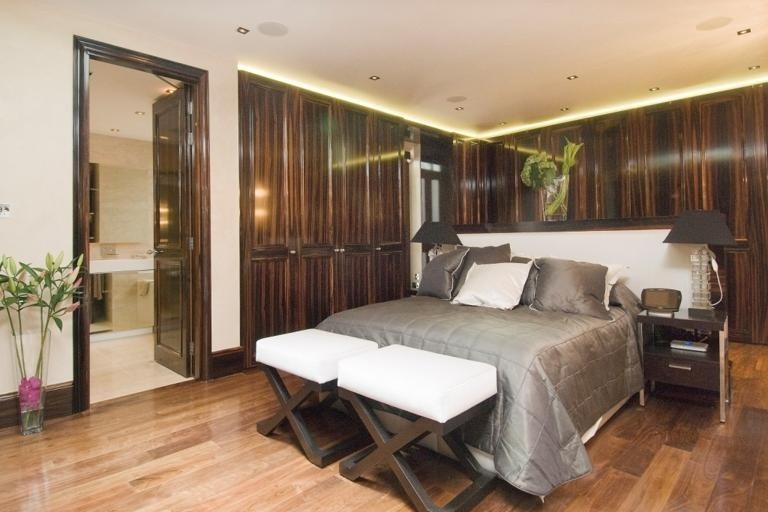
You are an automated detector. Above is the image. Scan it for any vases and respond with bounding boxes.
[9,325,52,436]
[538,174,569,222]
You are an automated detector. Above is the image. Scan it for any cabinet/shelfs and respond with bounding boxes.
[89,163,100,243]
[298,87,372,330]
[239,71,297,369]
[373,110,410,304]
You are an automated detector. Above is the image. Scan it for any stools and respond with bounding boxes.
[254,329,378,469]
[333,343,498,512]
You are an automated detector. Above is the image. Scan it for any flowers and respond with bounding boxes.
[519,135,583,216]
[0,253,88,426]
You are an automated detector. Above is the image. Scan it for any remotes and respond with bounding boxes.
[671,340,708,352]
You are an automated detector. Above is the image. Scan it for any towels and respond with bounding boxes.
[139,280,154,296]
[93,274,103,300]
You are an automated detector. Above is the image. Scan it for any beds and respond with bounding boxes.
[313,216,709,497]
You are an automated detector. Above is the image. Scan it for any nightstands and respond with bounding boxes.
[635,308,731,423]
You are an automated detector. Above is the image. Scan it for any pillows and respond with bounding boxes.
[416,244,619,322]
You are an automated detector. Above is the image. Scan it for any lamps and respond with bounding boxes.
[410,222,463,258]
[662,208,739,317]
